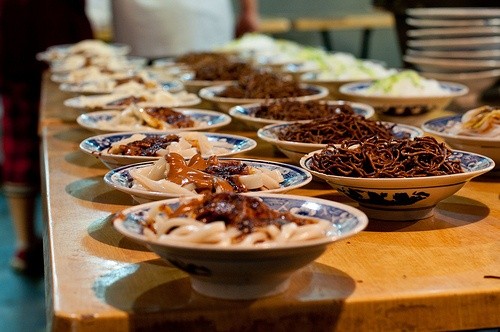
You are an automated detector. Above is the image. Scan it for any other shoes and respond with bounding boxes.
[10,234,43,270]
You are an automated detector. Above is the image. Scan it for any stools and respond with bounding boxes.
[257,9,395,59]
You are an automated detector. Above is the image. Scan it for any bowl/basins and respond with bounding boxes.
[76,110,232,131]
[402,7,500,108]
[102,161,313,207]
[256,122,424,181]
[202,41,387,101]
[299,144,496,222]
[37,42,202,108]
[227,99,375,129]
[421,114,500,169]
[111,193,369,299]
[338,80,469,123]
[79,132,257,170]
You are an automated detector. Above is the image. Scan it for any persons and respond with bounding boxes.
[113,0,261,61]
[0,0,94,278]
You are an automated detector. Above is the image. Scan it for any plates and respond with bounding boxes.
[198,83,330,114]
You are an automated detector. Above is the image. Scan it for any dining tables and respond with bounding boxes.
[37,69,500,332]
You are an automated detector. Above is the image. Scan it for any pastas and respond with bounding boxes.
[307,135,464,177]
[278,112,398,143]
[175,52,319,99]
[253,97,353,122]
